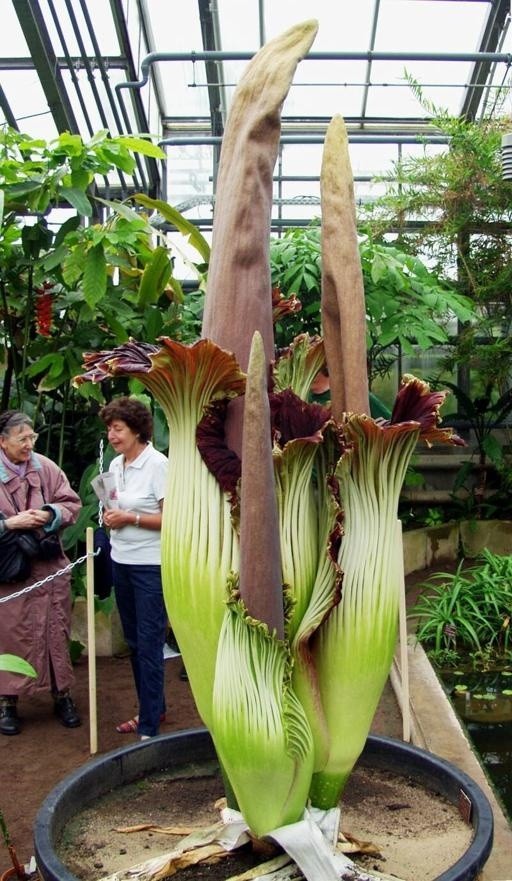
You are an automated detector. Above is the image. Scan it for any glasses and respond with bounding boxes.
[1,431,38,444]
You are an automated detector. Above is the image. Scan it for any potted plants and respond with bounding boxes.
[22,14,498,881]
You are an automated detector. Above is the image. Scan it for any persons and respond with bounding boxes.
[1,408,87,736]
[99,396,171,744]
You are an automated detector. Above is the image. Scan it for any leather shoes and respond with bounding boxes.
[1,699,21,735]
[54,698,82,727]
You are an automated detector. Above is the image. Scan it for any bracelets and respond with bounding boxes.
[133,514,144,528]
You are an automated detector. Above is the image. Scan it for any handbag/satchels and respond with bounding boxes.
[2,530,35,585]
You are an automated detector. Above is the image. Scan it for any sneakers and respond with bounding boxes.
[116,713,166,734]
